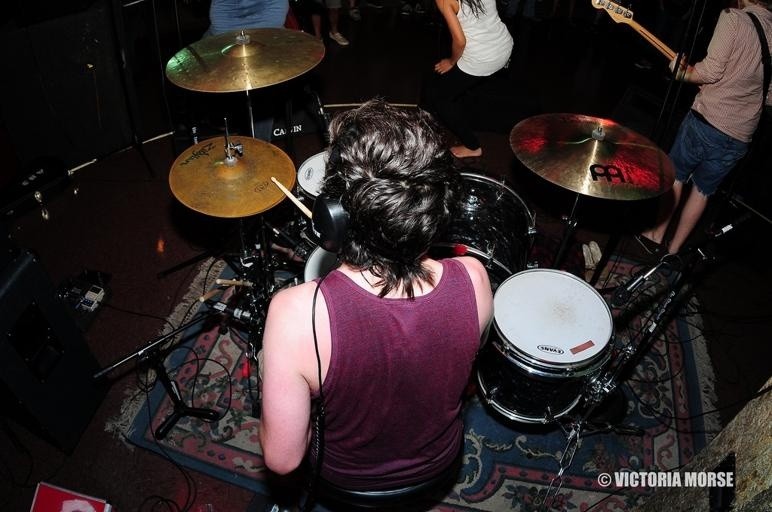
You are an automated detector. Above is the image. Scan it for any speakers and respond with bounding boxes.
[0,236,112,456]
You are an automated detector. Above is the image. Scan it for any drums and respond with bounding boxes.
[458,174,532,280]
[293,147,333,194]
[304,246,337,282]
[475,268,615,430]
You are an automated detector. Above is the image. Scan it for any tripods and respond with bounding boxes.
[139,349,220,436]
[204,220,303,418]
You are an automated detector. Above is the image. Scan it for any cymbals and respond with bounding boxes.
[168,26,325,99]
[168,134,295,220]
[510,114,675,202]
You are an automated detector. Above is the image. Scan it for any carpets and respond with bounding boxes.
[107,243,709,511]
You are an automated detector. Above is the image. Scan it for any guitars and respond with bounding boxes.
[593,0,677,64]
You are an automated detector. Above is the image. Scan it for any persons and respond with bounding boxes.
[638,0,771,255]
[427,1,515,159]
[400,0,426,16]
[258,97,495,512]
[202,0,362,128]
